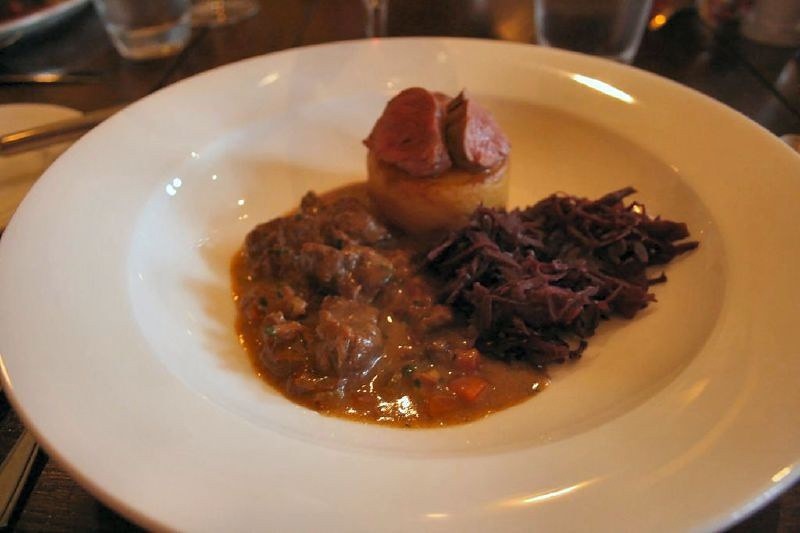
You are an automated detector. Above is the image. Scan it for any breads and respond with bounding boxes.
[364,148,510,233]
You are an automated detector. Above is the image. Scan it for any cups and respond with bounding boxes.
[92,0,192,61]
[534,0,654,65]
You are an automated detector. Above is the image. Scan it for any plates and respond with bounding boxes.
[1,37,800,533]
[0,0,90,38]
[0,103,87,233]
